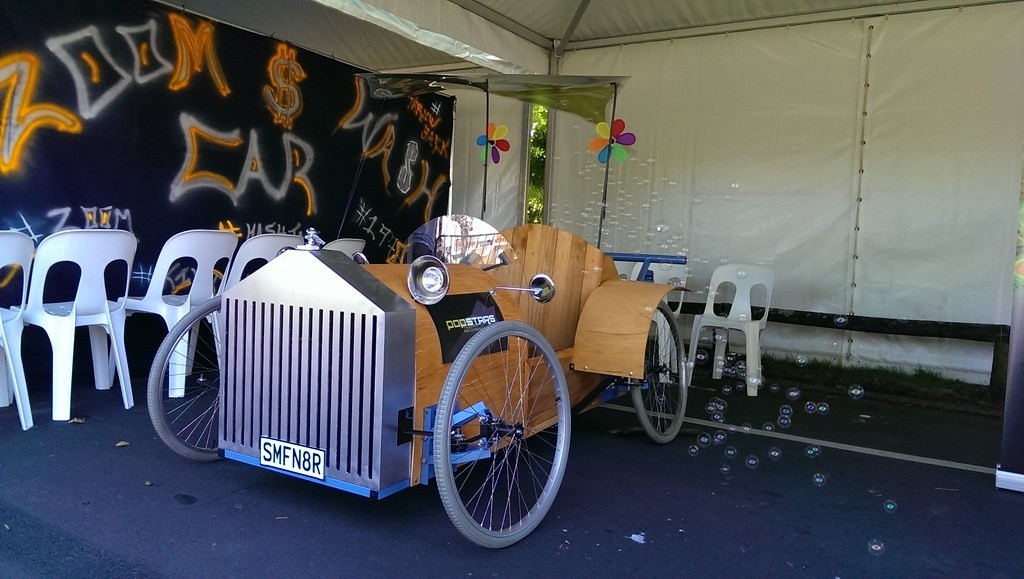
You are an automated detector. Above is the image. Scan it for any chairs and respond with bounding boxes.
[0,228,774,430]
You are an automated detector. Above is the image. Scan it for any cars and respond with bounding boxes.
[146,223,689,549]
